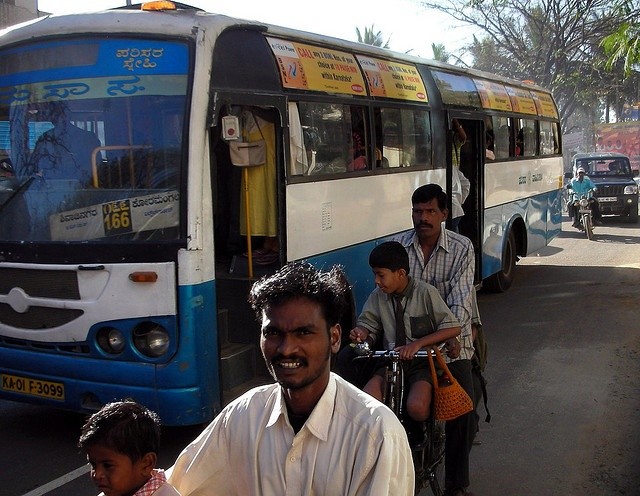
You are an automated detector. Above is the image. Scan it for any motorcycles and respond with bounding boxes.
[566,184,601,240]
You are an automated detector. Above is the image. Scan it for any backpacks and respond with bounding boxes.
[473,325,490,423]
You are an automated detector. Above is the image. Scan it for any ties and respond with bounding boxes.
[393,295,406,348]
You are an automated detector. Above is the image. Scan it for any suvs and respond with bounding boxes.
[565,151,638,223]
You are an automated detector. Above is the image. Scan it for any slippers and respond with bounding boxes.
[243,250,263,257]
[256,254,279,265]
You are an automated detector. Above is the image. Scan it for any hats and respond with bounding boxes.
[577,168,585,173]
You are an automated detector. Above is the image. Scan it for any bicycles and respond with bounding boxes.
[348,340,482,496]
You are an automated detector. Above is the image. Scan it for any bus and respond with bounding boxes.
[0,0,565,427]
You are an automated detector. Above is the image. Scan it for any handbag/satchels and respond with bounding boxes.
[424,345,474,421]
[229,110,266,167]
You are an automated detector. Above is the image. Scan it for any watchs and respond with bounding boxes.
[456,125,463,129]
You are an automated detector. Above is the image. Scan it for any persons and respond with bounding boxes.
[349,241,461,423]
[161,258,417,496]
[299,118,431,173]
[452,166,471,235]
[27,101,102,184]
[389,183,475,496]
[607,162,624,174]
[451,119,467,170]
[236,105,280,264]
[77,398,182,496]
[471,284,482,453]
[568,167,602,225]
